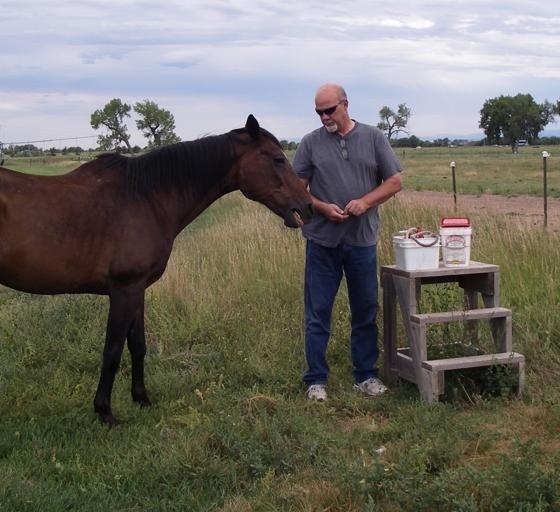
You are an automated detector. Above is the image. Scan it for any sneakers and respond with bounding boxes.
[305,384,329,402]
[355,377,391,397]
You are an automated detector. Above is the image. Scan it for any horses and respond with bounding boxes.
[0,114,313,430]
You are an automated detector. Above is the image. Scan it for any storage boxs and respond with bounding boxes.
[440,216,472,267]
[392,235,440,270]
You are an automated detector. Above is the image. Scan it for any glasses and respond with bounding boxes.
[315,98,345,116]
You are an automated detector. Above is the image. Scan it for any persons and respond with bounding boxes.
[291,84,403,402]
